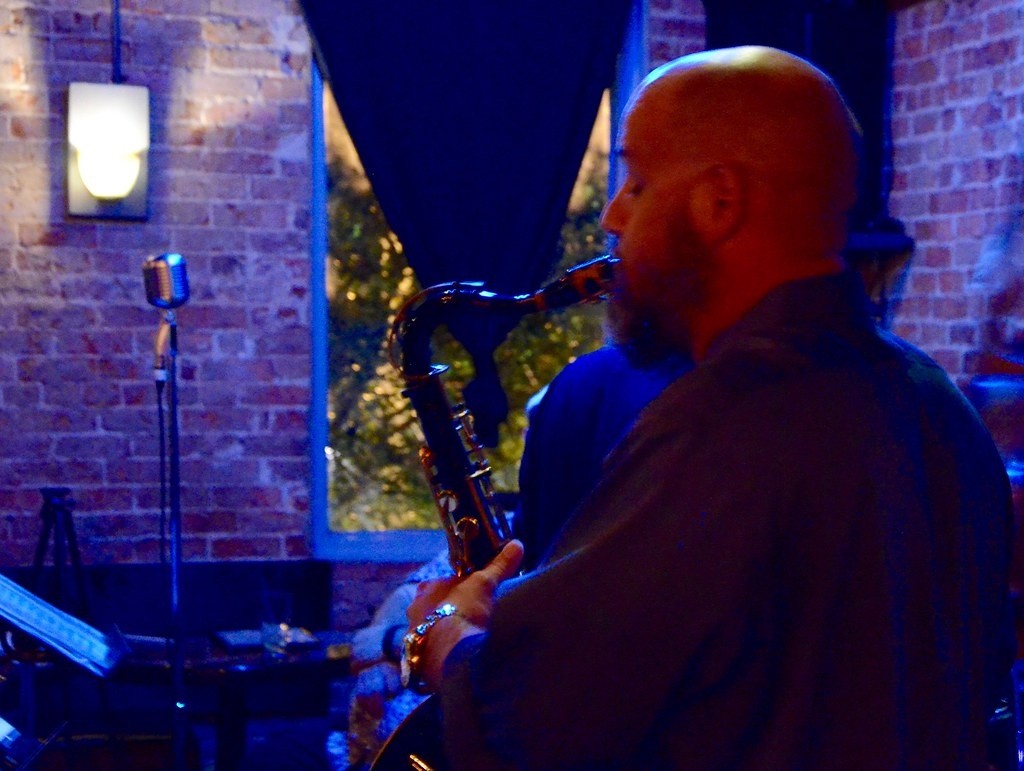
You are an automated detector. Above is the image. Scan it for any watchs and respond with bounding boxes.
[399,596,459,694]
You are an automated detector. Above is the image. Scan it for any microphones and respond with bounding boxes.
[142,251,190,309]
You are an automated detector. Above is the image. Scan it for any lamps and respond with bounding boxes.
[65,82,151,221]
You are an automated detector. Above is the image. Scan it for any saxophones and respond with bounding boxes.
[368,255,640,770]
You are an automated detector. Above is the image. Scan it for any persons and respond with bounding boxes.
[507,320,695,577]
[389,43,1023,771]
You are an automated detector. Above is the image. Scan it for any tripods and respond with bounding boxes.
[20,485,132,771]
